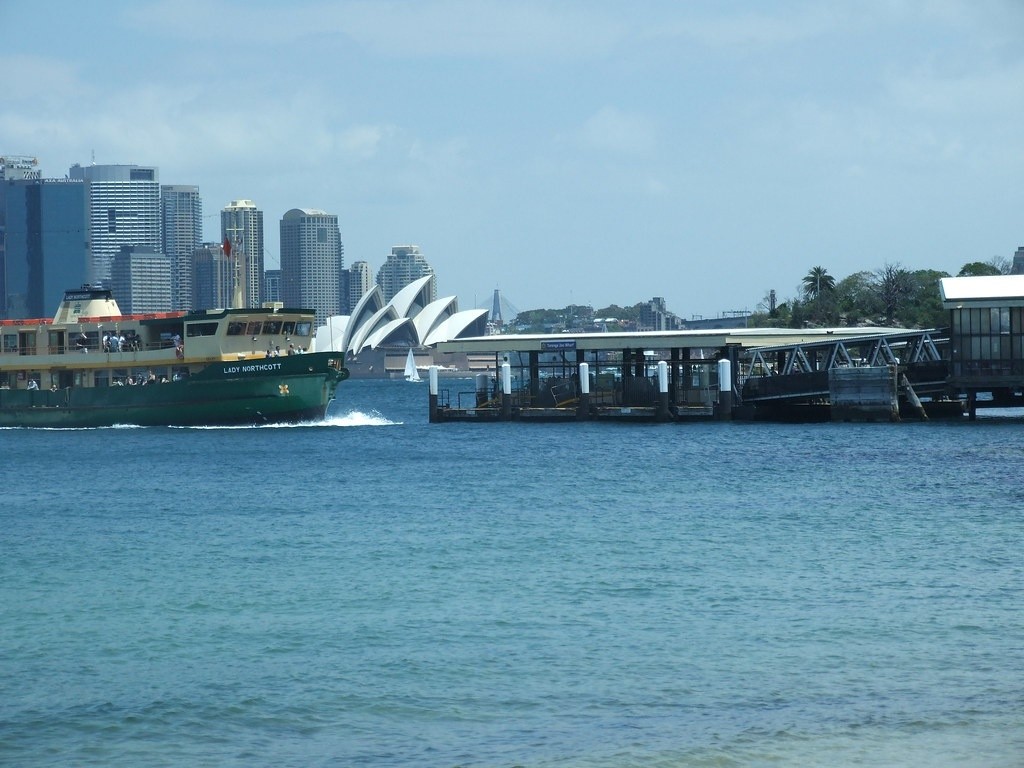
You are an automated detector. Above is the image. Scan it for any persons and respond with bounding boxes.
[288,343,302,356]
[25,378,39,391]
[76,332,88,354]
[171,332,180,347]
[265,345,281,358]
[50,385,58,393]
[102,331,141,353]
[111,369,183,387]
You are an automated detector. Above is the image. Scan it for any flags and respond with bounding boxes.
[222,237,231,262]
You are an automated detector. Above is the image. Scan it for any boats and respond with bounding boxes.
[0,282,350,426]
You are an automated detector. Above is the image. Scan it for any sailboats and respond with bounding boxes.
[403,348,428,382]
